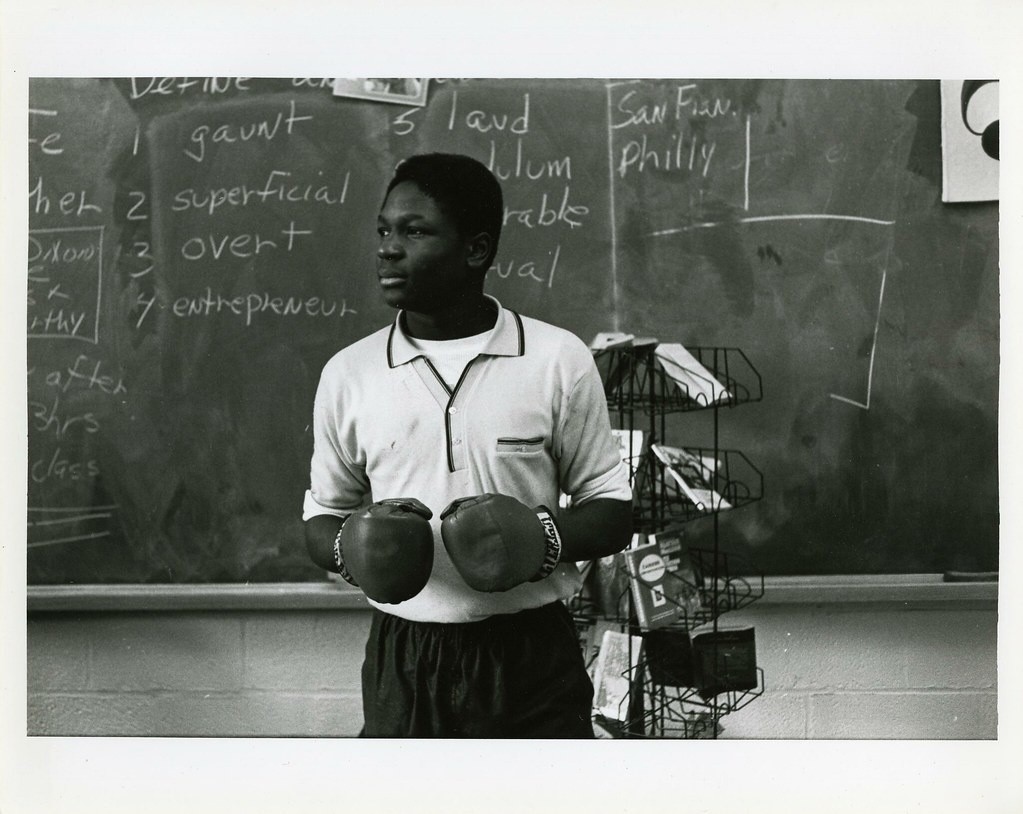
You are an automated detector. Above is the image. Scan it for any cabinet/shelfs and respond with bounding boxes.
[566,343,767,738]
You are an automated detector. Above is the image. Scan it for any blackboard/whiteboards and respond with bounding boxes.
[28,79,999,611]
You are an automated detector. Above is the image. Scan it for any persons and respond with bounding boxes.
[300,154,633,739]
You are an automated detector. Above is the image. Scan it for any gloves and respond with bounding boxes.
[334,498,435,604]
[440,494,563,593]
